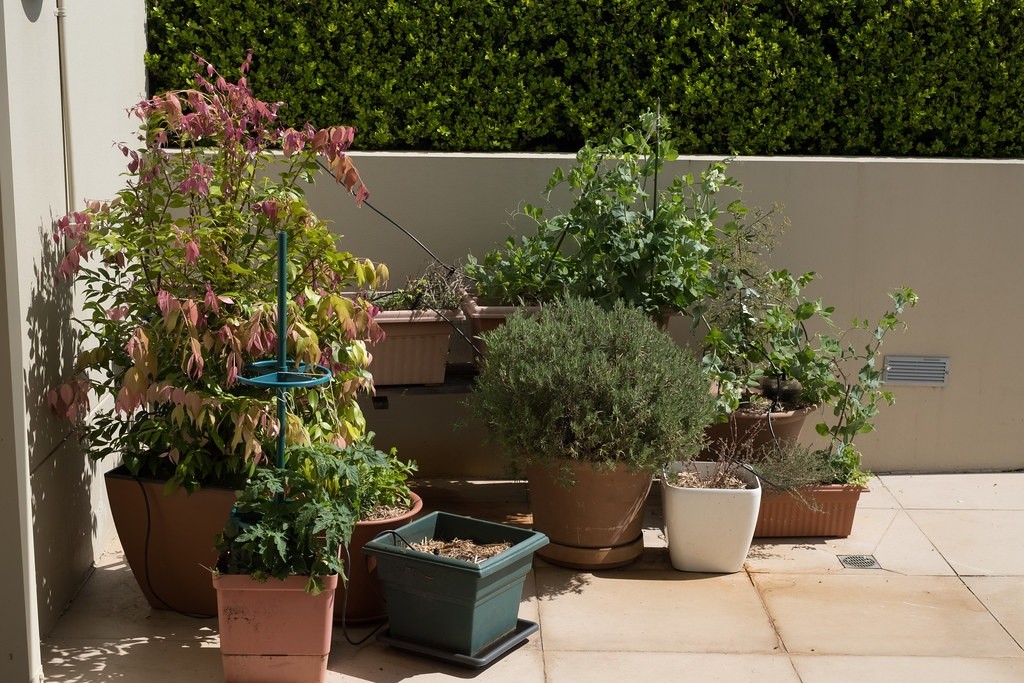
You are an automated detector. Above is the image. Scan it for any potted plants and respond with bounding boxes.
[41,54,919,683]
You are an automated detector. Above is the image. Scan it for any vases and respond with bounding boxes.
[360,510,549,671]
